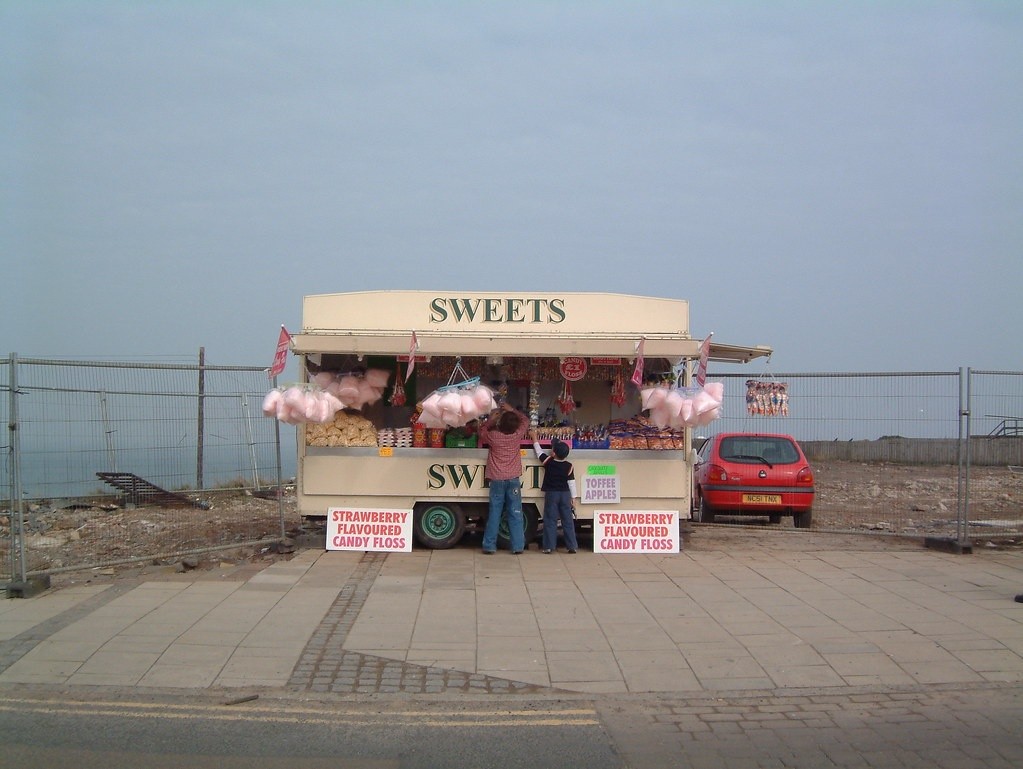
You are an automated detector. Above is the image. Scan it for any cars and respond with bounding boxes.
[692,432,816,529]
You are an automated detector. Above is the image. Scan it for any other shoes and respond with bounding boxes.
[543,548,551,554]
[482,550,495,554]
[512,549,523,554]
[567,547,577,553]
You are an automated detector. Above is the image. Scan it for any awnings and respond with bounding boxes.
[290,334,705,356]
[710,342,774,363]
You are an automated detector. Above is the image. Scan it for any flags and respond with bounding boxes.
[695,337,710,387]
[631,339,645,385]
[405,333,418,383]
[269,329,291,379]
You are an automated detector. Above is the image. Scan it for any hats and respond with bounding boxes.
[551,437,569,457]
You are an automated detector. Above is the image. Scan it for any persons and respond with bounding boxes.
[477,403,530,554]
[528,430,578,554]
